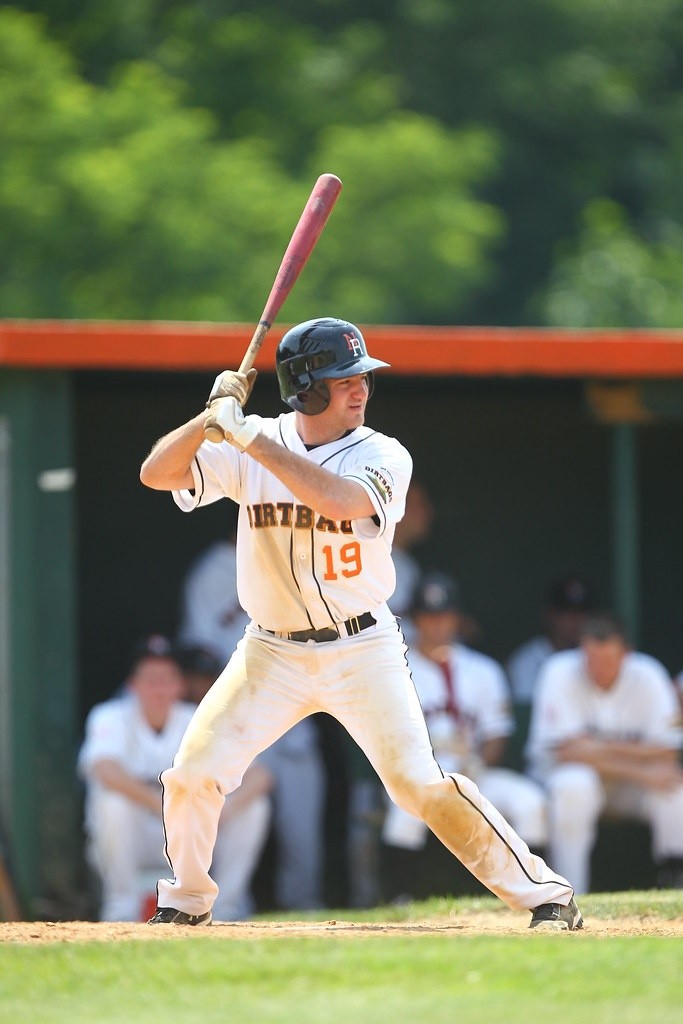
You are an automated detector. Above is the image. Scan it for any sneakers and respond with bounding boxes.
[145,906,212,929]
[530,897,585,934]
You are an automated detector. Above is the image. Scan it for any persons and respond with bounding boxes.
[376,574,550,903]
[140,317,583,931]
[75,635,276,922]
[174,484,613,921]
[525,614,683,896]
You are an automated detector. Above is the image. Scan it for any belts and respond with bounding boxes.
[256,613,380,645]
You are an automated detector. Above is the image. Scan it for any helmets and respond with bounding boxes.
[273,317,391,415]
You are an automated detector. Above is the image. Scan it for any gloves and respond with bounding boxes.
[204,369,266,456]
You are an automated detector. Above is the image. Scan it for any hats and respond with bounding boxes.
[131,630,181,674]
[409,571,465,613]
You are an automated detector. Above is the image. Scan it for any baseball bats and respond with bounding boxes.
[203,170,344,445]
